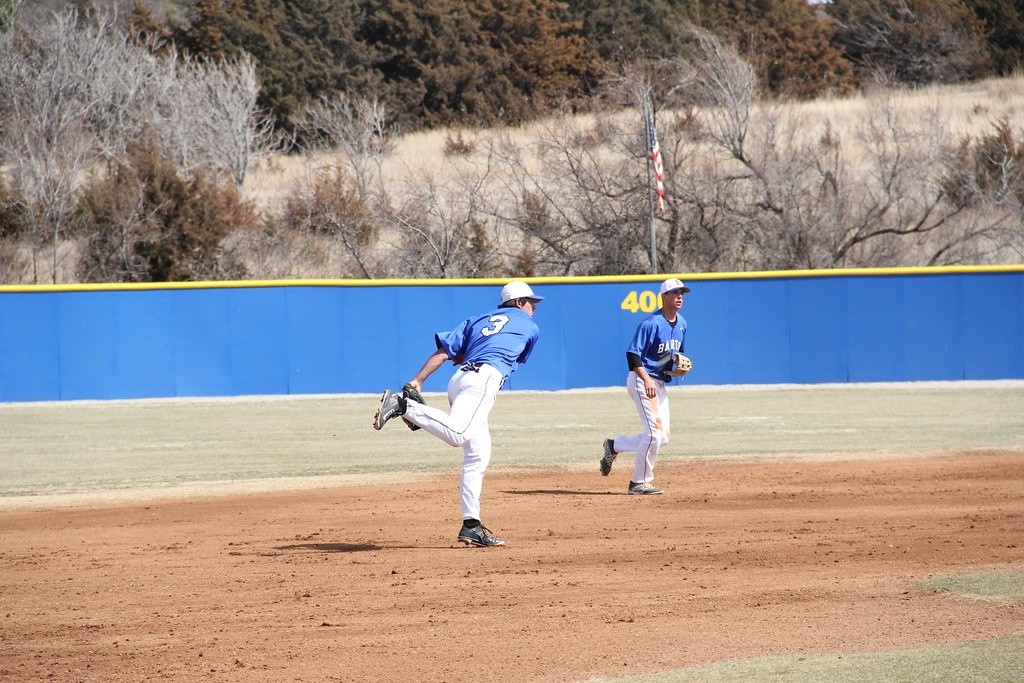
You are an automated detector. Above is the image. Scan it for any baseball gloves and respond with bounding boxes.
[400,382,428,431]
[663,352,694,377]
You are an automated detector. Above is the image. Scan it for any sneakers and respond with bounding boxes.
[457,524,505,548]
[373,389,400,431]
[600,438,618,476]
[628,481,664,495]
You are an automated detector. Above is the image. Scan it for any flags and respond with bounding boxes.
[647,111,665,213]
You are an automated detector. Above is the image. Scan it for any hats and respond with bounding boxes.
[500,281,545,303]
[660,278,691,293]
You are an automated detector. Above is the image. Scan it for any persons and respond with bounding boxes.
[373,281,545,547]
[601,278,691,495]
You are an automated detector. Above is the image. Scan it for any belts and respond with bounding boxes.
[473,363,484,367]
